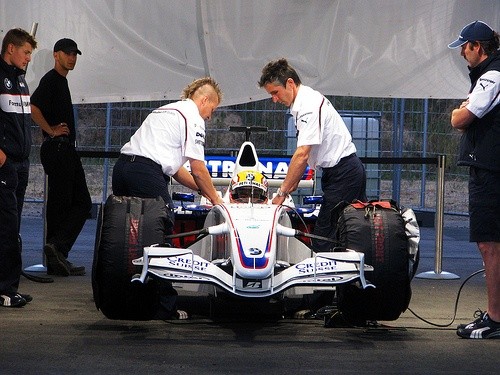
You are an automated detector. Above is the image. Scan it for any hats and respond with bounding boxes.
[448,20,494,49]
[54,37,82,55]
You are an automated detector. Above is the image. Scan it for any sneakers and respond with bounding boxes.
[457,308,500,339]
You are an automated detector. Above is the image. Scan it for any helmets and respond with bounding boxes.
[230,170,269,193]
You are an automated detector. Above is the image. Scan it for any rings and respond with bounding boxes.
[60,122,63,125]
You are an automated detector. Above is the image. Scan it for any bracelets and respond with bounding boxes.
[49,127,56,138]
[197,189,202,195]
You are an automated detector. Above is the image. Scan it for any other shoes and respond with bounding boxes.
[156,309,190,320]
[294,302,332,320]
[16,292,33,302]
[0,294,26,307]
[47,261,86,276]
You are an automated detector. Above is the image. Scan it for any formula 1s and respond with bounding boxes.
[90,125,421,322]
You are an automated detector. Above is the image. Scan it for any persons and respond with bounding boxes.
[0,28,35,308]
[111,77,226,322]
[27,38,92,276]
[257,57,370,321]
[450,20,500,338]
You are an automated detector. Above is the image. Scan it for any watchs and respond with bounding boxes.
[276,188,288,198]
